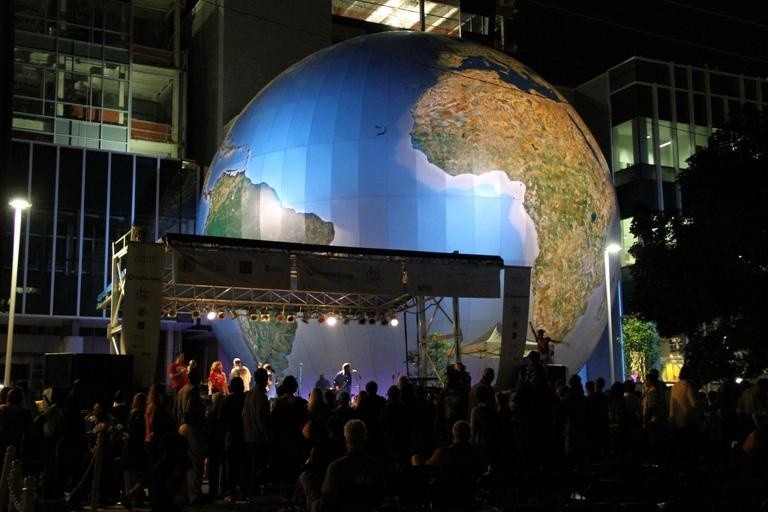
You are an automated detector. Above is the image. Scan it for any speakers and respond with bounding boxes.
[521,364,566,385]
[42,351,133,391]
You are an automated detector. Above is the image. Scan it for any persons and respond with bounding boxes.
[529,320,569,365]
[0,350,768,511]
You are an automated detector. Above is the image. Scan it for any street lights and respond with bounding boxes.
[4,193,32,387]
[603,237,622,387]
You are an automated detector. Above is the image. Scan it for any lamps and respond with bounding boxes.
[160,284,404,326]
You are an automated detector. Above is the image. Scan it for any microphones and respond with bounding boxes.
[352,369,358,373]
